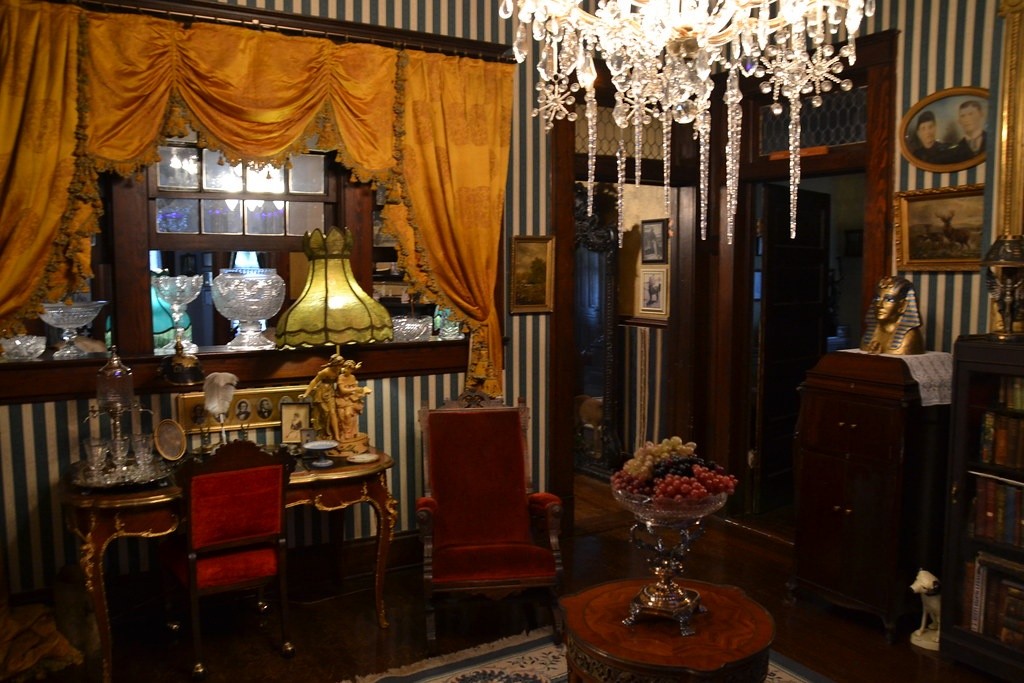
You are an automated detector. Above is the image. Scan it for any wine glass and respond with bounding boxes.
[152,274,204,355]
[82,433,153,482]
[34,300,109,359]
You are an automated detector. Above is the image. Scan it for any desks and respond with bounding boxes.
[564,576,778,683]
[57,437,394,683]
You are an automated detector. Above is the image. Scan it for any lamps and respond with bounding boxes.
[277,227,395,457]
[498,0,877,246]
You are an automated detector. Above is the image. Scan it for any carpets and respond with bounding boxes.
[336,622,837,683]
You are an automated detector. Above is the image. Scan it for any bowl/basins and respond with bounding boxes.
[392,315,433,342]
[0,336,46,359]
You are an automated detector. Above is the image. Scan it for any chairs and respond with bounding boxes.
[145,435,299,681]
[416,396,566,652]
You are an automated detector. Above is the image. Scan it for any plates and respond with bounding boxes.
[346,454,379,463]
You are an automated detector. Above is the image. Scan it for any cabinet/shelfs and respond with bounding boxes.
[784,350,954,647]
[938,333,1024,683]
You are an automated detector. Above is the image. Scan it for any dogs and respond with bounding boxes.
[909,568,940,642]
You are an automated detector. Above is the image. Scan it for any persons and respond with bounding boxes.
[651,229,658,255]
[648,277,661,301]
[298,354,365,440]
[913,100,987,164]
[861,278,925,355]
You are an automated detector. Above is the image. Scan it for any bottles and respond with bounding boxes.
[439,306,465,341]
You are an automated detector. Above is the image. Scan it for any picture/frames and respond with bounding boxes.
[893,182,985,273]
[640,268,669,314]
[279,402,311,445]
[899,85,992,174]
[641,218,669,264]
[509,234,556,314]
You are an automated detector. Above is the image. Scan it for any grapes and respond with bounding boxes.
[611,435,739,515]
